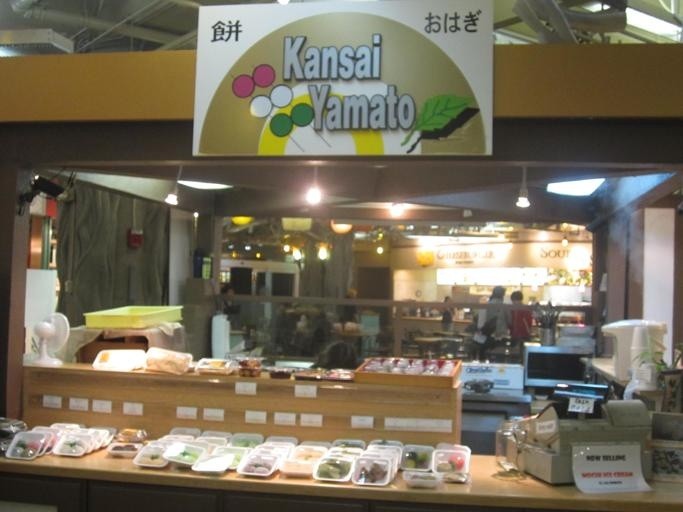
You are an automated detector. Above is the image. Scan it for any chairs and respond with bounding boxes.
[369,328,395,358]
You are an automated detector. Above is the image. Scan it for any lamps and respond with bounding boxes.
[161,163,184,206]
[416,247,435,269]
[513,166,529,208]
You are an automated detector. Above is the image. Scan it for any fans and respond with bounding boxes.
[32,312,69,368]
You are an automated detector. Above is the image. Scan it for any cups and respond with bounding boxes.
[629,327,653,383]
[539,328,555,346]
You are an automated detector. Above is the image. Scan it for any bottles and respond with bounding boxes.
[494,425,527,480]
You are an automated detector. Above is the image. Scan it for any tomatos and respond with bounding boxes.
[448,454,464,470]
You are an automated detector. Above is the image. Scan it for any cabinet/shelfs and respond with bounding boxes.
[19,357,464,449]
[75,321,183,363]
[0,428,680,512]
[580,356,667,413]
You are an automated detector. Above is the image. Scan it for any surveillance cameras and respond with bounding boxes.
[30,175,67,199]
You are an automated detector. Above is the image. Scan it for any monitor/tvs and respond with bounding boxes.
[522,344,595,392]
[255,272,293,296]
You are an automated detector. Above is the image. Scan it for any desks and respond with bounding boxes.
[415,336,462,358]
[335,329,373,363]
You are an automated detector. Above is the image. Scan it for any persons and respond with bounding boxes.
[438,296,455,334]
[220,285,233,314]
[506,290,532,347]
[481,285,511,342]
[272,305,294,334]
[309,340,363,370]
[342,289,358,324]
[291,304,310,323]
[303,304,326,342]
